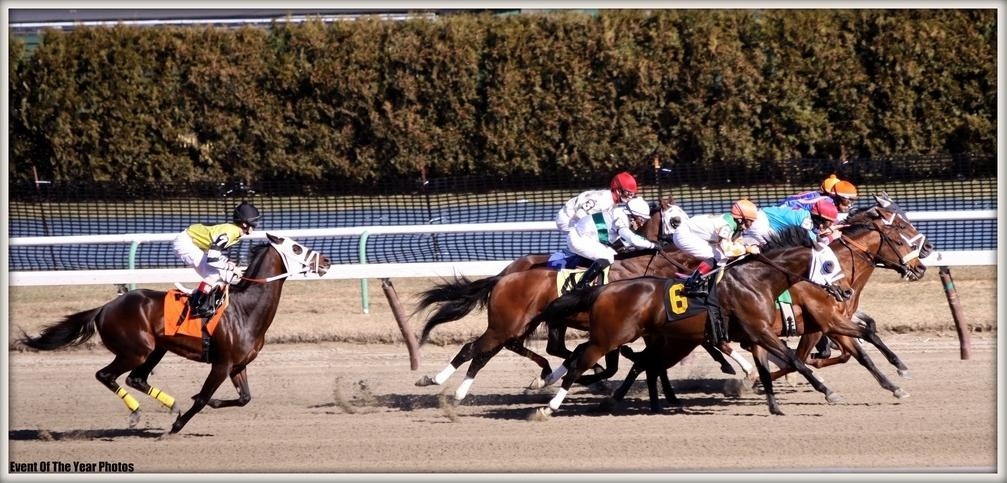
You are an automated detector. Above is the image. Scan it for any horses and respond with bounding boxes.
[405,191,933,421]
[9,233,331,435]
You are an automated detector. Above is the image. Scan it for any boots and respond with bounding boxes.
[572,262,603,288]
[680,270,709,296]
[191,290,215,317]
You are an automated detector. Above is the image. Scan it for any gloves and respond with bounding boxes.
[232,266,243,279]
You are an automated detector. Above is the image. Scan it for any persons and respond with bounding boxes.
[171,200,261,290]
[555,168,861,290]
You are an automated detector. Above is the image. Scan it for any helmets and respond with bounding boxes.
[624,197,651,220]
[811,174,857,221]
[233,201,261,225]
[731,199,759,221]
[611,172,640,192]
